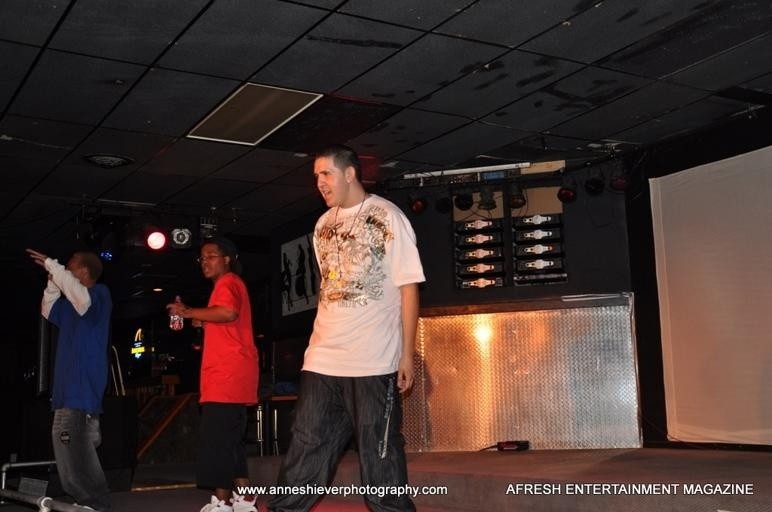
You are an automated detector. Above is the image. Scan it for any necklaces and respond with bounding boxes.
[334,191,367,279]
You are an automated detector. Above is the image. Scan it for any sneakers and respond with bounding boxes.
[199,495,233,512]
[230,491,259,512]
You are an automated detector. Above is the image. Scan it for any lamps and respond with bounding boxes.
[404,143,648,214]
[65,198,250,250]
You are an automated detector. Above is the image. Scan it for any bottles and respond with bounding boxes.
[169,295,184,330]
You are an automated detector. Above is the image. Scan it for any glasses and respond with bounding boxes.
[196,253,227,262]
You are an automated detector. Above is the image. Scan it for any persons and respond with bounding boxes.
[164,238,261,511]
[262,142,428,512]
[26,246,115,511]
